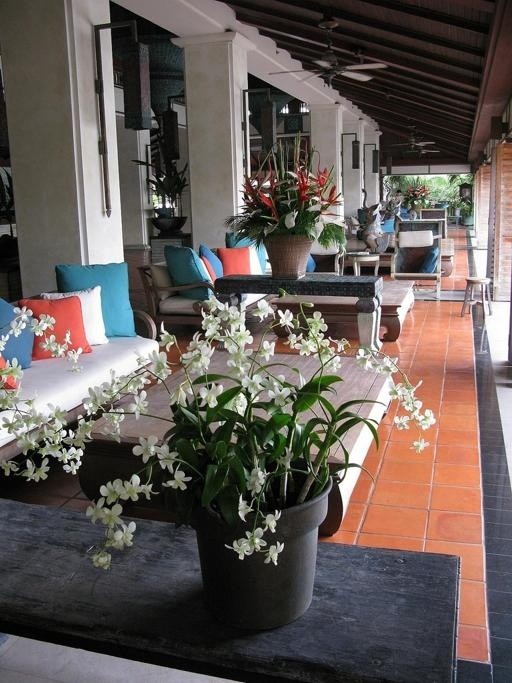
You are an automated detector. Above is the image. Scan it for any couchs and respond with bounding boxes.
[0,290,160,476]
[138,260,271,335]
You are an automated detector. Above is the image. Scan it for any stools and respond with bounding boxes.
[350,252,380,277]
[461,276,493,318]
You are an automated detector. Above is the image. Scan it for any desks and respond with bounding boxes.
[1,496,461,683]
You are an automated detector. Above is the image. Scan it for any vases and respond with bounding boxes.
[413,205,424,219]
[185,478,333,629]
[263,234,312,280]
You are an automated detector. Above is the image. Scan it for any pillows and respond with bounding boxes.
[157,232,266,301]
[420,248,440,273]
[1,263,139,389]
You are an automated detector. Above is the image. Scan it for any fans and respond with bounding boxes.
[388,130,437,151]
[266,37,390,87]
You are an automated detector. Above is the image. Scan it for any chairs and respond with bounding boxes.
[390,231,441,300]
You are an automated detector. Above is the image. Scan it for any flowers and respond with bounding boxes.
[224,131,348,249]
[0,289,437,565]
[404,178,431,204]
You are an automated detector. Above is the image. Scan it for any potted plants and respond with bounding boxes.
[460,204,473,226]
[130,134,189,233]
